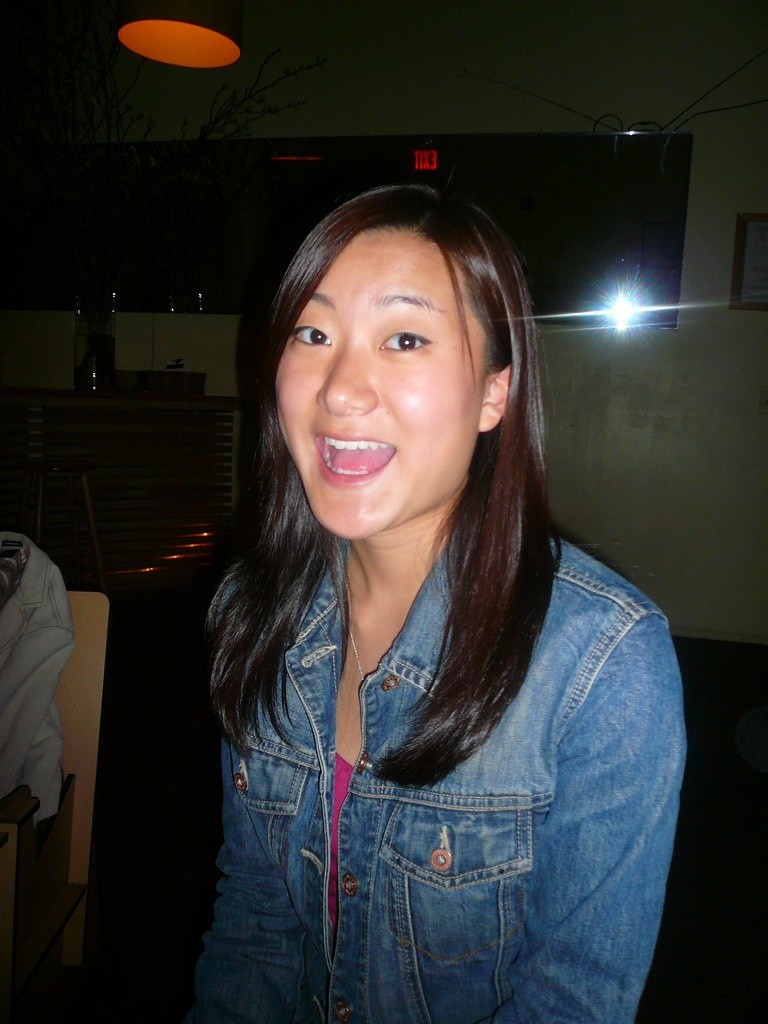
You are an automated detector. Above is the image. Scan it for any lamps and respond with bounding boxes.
[117,0,244,69]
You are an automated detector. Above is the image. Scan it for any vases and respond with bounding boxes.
[72,287,118,397]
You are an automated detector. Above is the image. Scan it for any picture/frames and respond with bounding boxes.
[730,213,768,310]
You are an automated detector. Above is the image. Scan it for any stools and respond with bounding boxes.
[15,459,112,629]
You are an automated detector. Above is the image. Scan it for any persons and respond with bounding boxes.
[196,179,685,1023]
[1,524,71,984]
